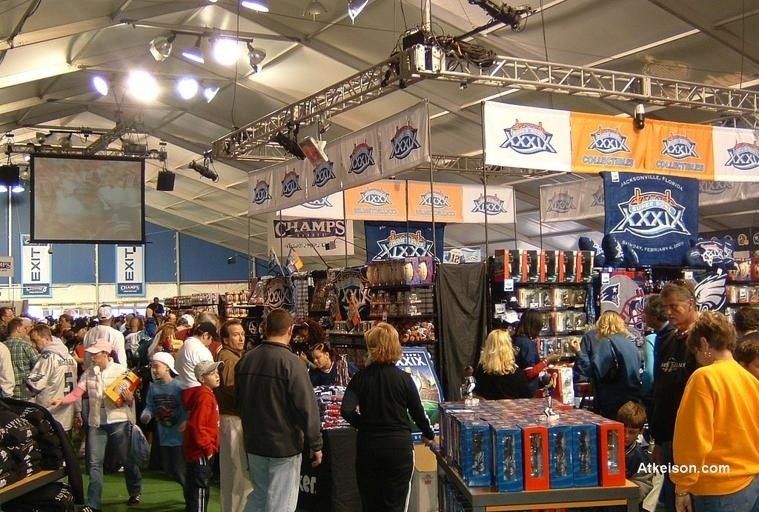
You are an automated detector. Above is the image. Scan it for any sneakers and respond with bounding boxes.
[128,494,141,505]
[76,505,100,512]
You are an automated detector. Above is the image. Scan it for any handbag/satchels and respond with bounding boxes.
[130,425,150,469]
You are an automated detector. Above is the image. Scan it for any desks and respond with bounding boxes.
[1,466,72,511]
[427,441,641,512]
[413,444,439,512]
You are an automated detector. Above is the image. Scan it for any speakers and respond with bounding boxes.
[156,171,175,191]
[0,165,19,186]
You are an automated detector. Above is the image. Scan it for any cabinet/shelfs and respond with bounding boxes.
[488,282,595,368]
[724,279,759,308]
[166,303,256,324]
[361,283,438,343]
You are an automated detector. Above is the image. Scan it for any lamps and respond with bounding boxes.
[1,0,648,270]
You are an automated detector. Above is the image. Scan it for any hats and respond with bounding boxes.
[153,352,179,376]
[195,361,225,379]
[85,338,111,353]
[177,314,194,327]
[98,305,112,320]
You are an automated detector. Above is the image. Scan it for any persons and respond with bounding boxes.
[541,372,558,417]
[460,366,476,399]
[510,309,558,390]
[0,297,350,512]
[570,279,759,512]
[479,328,535,398]
[340,323,437,512]
